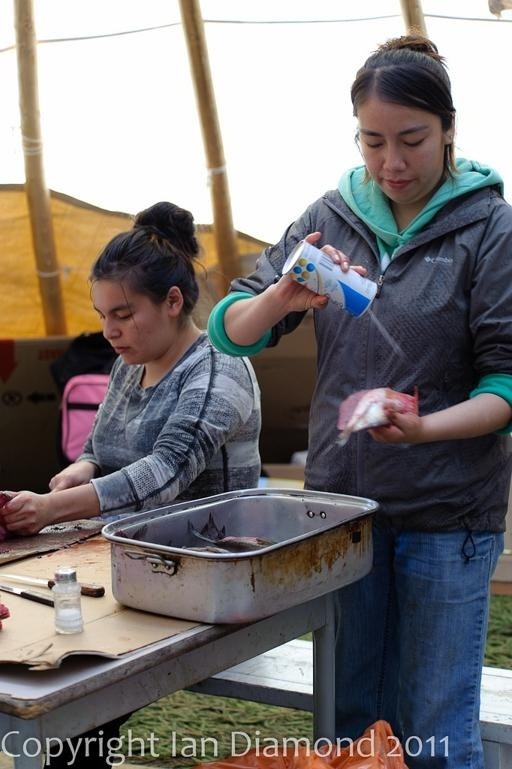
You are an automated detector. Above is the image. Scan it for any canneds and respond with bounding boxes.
[280,239,379,321]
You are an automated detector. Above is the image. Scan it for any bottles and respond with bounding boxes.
[54,568,85,634]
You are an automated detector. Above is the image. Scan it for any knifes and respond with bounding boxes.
[1,574,105,598]
[0,582,56,609]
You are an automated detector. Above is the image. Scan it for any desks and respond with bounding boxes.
[0,518,334,769]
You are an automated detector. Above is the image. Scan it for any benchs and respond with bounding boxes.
[184,639,512,769]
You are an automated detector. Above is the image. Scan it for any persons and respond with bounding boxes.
[204,31,512,769]
[2,198,261,769]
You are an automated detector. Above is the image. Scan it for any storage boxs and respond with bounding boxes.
[259,462,305,490]
[490,549,512,596]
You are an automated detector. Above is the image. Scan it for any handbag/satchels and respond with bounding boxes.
[61,373,110,464]
